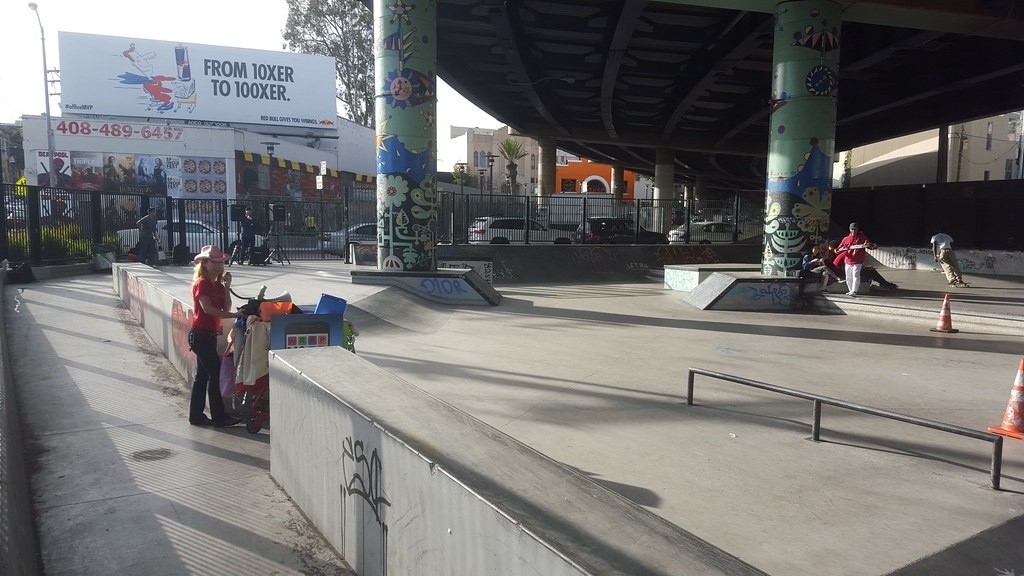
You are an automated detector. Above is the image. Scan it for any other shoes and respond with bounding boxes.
[846,291,852,295]
[849,292,856,297]
[949,280,956,285]
[821,288,830,294]
[212,414,243,426]
[958,281,964,283]
[189,414,215,426]
[837,278,845,283]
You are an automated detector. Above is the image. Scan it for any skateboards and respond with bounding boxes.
[946,283,972,288]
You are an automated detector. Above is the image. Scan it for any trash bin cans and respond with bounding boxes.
[91,243,118,274]
[172,244,190,266]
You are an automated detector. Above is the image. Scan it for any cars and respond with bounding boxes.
[667,220,746,244]
[116,217,266,260]
[317,222,377,258]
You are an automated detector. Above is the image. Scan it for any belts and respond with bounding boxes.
[190,328,217,336]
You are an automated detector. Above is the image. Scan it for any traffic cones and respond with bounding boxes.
[929,293,959,333]
[989,356,1024,441]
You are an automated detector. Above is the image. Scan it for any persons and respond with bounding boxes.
[136,207,158,267]
[154,199,166,220]
[122,155,135,182]
[834,223,877,296]
[819,240,899,289]
[188,244,245,427]
[239,207,255,265]
[930,228,969,288]
[106,198,137,223]
[138,158,149,180]
[802,244,846,293]
[154,158,165,182]
[103,156,118,180]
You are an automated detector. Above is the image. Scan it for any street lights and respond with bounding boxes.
[483,154,500,218]
[456,163,469,245]
[476,168,487,217]
[27,0,58,202]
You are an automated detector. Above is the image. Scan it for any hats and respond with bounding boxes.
[193,245,230,264]
[849,223,858,229]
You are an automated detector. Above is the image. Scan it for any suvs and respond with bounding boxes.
[467,216,572,245]
[572,216,670,245]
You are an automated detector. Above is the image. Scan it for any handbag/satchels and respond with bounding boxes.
[219,339,238,399]
